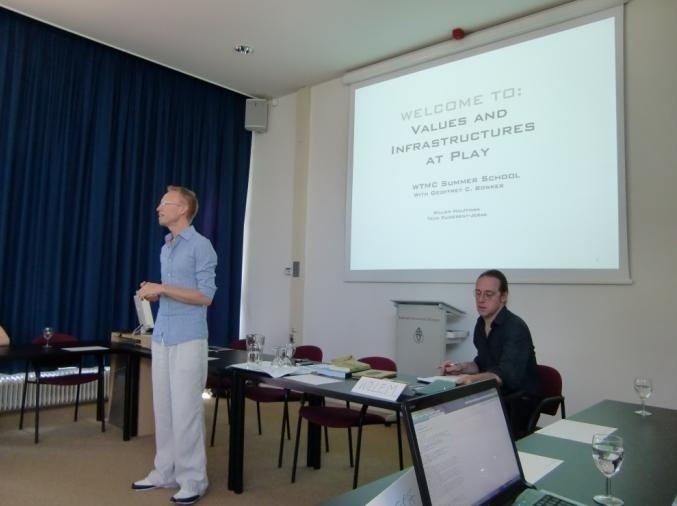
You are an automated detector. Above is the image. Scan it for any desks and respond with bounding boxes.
[112,340,316,494]
[231,356,429,496]
[0,341,111,433]
[314,399,675,506]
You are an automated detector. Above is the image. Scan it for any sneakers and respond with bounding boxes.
[129,469,179,492]
[170,487,206,505]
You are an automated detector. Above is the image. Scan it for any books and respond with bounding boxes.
[316,354,397,382]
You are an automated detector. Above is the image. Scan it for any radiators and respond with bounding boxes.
[0,367,110,415]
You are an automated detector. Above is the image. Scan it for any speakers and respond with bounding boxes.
[245,99,267,132]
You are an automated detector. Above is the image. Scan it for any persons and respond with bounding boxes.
[433,269,540,440]
[125,183,217,506]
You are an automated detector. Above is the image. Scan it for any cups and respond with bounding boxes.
[245,332,265,367]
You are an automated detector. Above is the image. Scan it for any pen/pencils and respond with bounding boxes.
[435,364,457,369]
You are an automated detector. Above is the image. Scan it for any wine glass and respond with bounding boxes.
[632,375,654,416]
[271,343,294,371]
[41,326,54,348]
[592,432,626,506]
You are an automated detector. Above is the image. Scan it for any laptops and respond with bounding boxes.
[401,377,587,506]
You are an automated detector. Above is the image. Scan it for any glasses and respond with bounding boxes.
[161,199,185,206]
[472,289,503,299]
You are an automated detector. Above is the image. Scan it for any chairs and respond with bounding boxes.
[18,332,106,445]
[290,356,405,490]
[209,339,258,448]
[243,343,322,469]
[505,363,566,444]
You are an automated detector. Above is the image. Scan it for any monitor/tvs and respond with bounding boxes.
[133,290,155,334]
